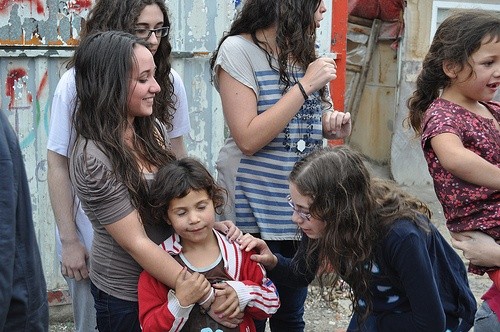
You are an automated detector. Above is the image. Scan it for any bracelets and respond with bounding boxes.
[295,81,308,100]
[199,287,216,314]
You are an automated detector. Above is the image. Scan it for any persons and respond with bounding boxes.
[46,0,352,332]
[0,109,50,332]
[406,9,500,332]
[239,145,477,332]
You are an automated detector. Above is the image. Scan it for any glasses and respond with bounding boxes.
[286,194,311,221]
[133,26,170,39]
